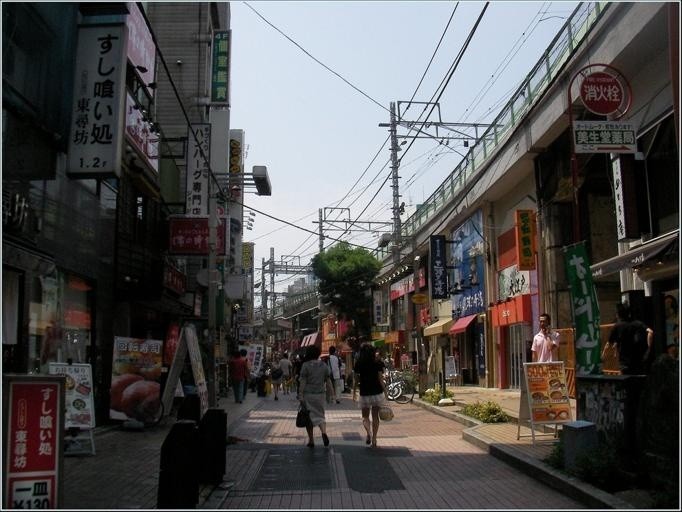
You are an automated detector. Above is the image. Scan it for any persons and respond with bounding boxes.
[599,302,653,374]
[296,346,338,448]
[231,345,347,404]
[530,312,561,363]
[351,342,390,447]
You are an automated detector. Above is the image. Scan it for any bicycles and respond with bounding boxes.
[384,367,415,404]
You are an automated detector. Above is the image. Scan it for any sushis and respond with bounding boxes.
[110,337,160,418]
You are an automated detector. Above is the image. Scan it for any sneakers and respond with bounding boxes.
[283,392,286,394]
[307,440,315,446]
[288,392,290,394]
[337,400,340,404]
[235,400,237,402]
[324,438,329,445]
[372,444,378,449]
[366,437,371,444]
[239,401,242,404]
[275,397,278,400]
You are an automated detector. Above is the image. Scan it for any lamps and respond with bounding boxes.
[243,208,256,230]
[376,232,417,249]
[210,164,277,198]
[127,64,157,110]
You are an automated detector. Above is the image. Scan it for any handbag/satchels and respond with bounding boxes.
[380,408,394,421]
[272,368,282,378]
[296,401,309,427]
